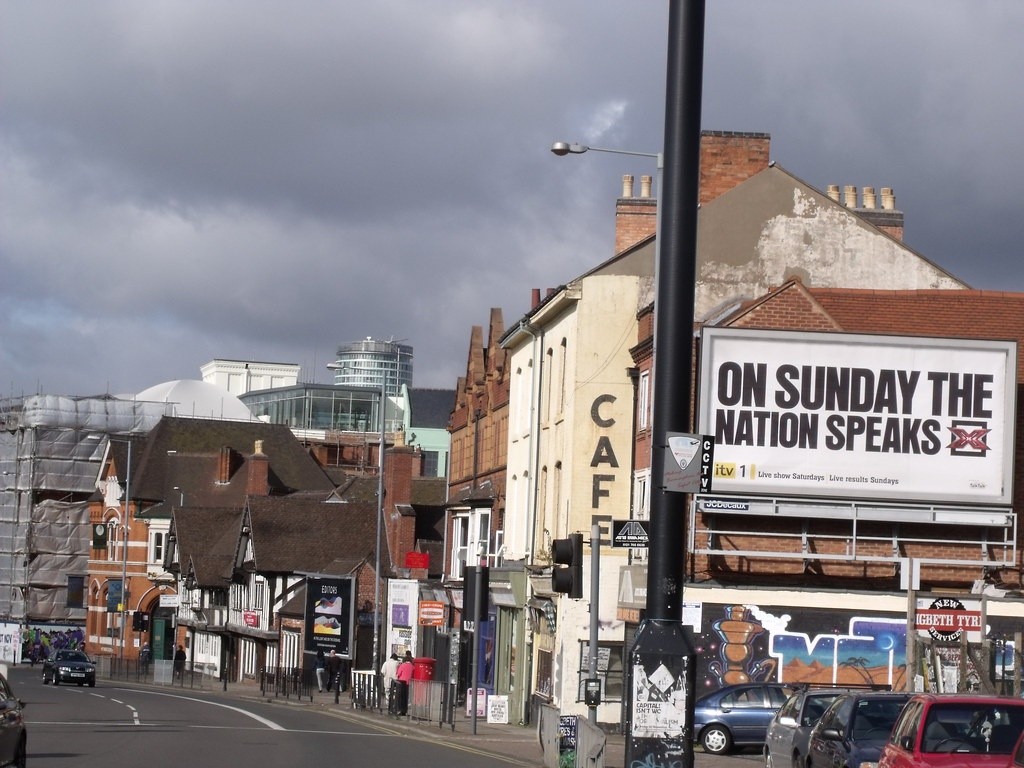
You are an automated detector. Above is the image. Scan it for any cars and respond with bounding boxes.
[762,686,857,768]
[42,649,98,687]
[691,681,809,755]
[0,674,28,768]
[806,691,929,767]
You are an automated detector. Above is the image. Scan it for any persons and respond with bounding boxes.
[381,650,415,716]
[139,642,152,674]
[312,650,341,692]
[174,645,186,679]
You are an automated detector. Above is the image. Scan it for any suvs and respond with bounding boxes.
[878,693,1024,768]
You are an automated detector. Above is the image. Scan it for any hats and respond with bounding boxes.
[144,642,148,646]
[331,650,335,652]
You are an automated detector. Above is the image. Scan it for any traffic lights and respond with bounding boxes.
[551,532,583,599]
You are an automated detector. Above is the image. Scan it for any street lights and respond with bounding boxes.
[553,141,658,436]
[324,364,388,679]
[88,434,131,668]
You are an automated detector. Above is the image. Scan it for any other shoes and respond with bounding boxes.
[319,689,322,693]
[396,711,401,715]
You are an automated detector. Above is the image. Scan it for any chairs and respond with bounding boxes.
[807,704,824,722]
[882,703,901,729]
[984,724,1020,754]
[926,720,959,752]
